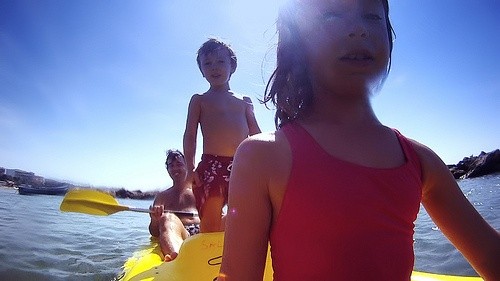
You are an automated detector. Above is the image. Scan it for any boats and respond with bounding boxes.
[18,183,69,195]
[113,233,484,281]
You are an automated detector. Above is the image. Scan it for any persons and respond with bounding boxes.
[148,150,227,262]
[213,0,499,280]
[181,39,264,232]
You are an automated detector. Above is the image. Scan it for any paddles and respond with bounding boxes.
[58,189,199,217]
